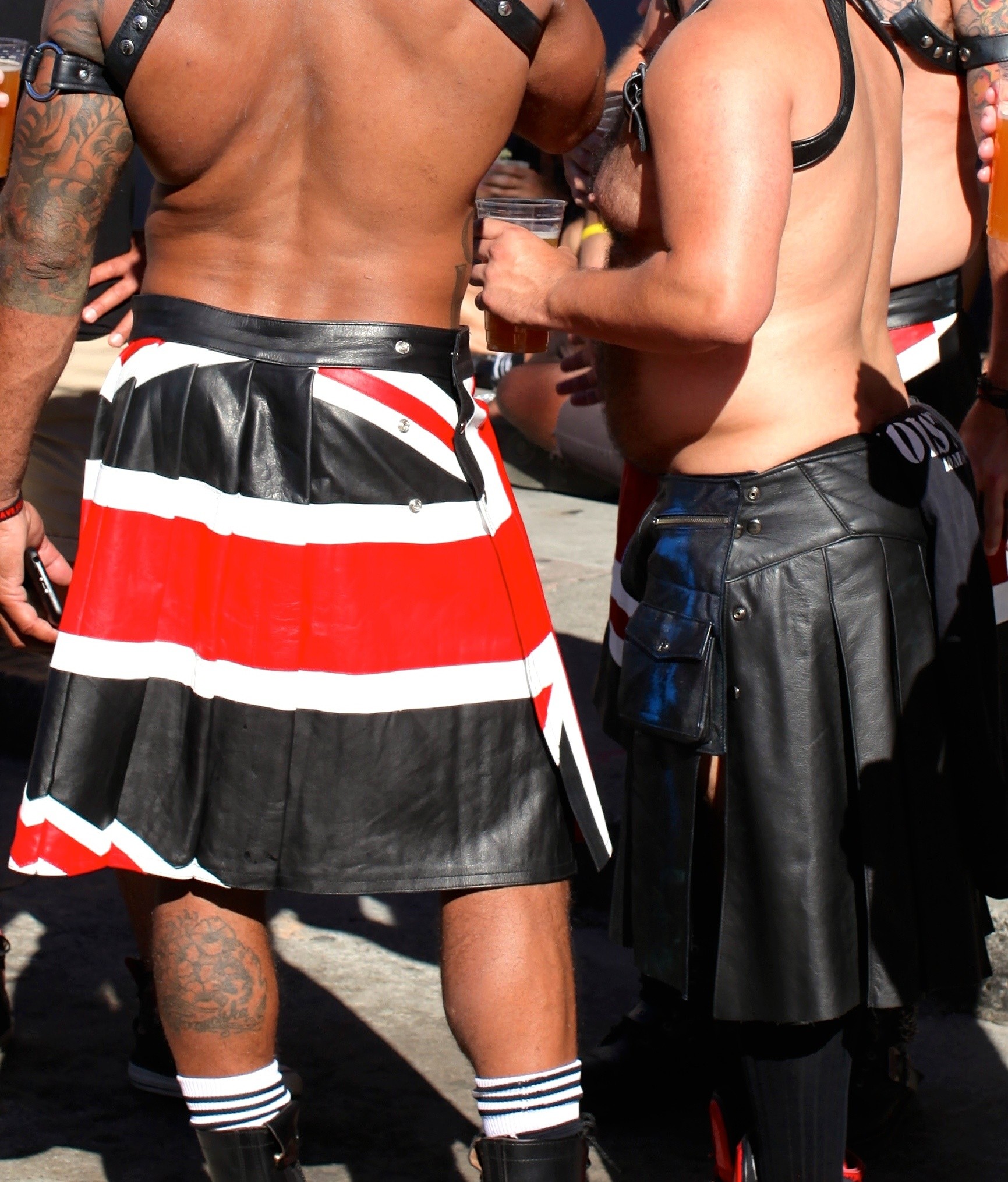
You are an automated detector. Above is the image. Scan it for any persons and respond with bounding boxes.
[0,0,1008,1182]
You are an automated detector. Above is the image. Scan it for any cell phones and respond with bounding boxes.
[20,547,63,630]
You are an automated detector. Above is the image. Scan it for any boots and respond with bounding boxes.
[196,1100,303,1182]
[475,1131,590,1182]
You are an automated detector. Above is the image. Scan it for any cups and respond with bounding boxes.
[475,200,572,355]
[985,66,1008,241]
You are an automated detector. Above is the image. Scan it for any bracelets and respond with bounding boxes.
[0,490,23,522]
[581,224,607,239]
[972,373,1008,407]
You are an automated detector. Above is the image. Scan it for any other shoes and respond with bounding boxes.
[125,1014,306,1101]
